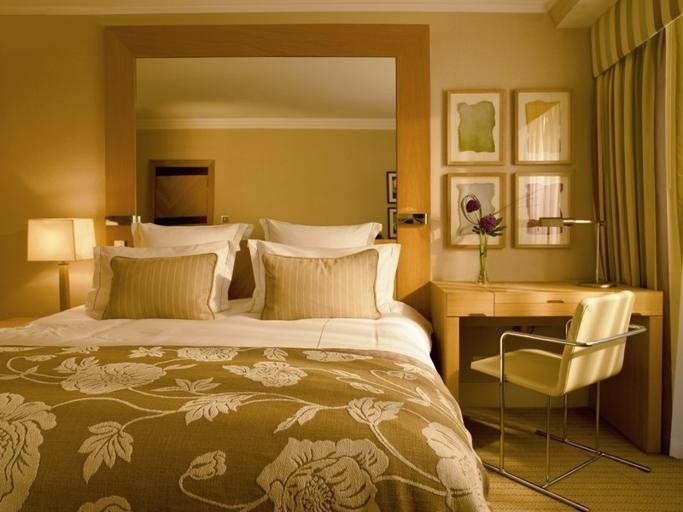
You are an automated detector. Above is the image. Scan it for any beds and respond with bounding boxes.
[0,220,491,512]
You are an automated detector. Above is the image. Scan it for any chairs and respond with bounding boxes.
[469,289,652,511]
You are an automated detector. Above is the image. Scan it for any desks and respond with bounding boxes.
[429,276,664,510]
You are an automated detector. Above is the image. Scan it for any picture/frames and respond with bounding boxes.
[386,207,398,239]
[385,171,397,205]
[444,171,508,251]
[512,87,576,166]
[444,88,509,167]
[512,169,574,251]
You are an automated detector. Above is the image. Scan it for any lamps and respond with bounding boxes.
[527,218,618,289]
[25,216,95,310]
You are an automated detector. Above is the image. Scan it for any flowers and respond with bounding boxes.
[460,193,502,246]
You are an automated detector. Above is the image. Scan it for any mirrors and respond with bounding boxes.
[131,55,397,241]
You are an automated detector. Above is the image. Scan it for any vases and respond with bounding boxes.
[476,247,489,287]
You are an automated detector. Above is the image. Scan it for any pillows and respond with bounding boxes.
[257,216,382,248]
[86,240,231,312]
[246,239,401,314]
[259,248,381,321]
[129,222,247,249]
[100,252,220,322]
